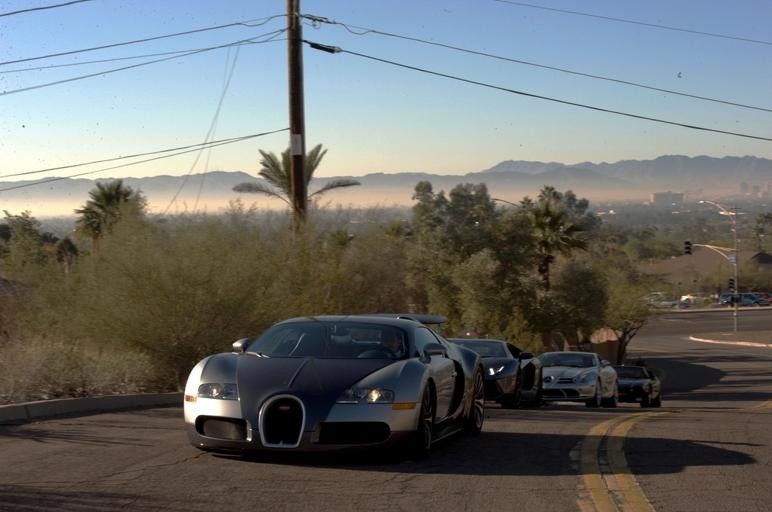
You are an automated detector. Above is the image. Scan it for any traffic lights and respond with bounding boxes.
[728,278,735,292]
[684,241,692,255]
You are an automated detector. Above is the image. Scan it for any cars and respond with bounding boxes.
[639,291,772,309]
[447,338,662,408]
[183,311,486,463]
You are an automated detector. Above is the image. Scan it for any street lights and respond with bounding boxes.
[699,200,738,334]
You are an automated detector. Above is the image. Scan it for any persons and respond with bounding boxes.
[377,328,402,358]
[477,344,493,358]
[576,356,594,368]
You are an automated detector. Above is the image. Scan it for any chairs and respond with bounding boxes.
[286,330,329,357]
